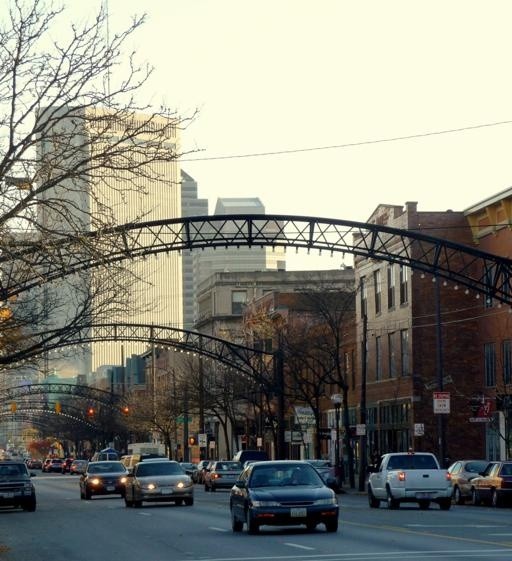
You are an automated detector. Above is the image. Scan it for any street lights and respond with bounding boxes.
[217,324,266,437]
[329,392,344,485]
[142,366,177,446]
[369,254,447,467]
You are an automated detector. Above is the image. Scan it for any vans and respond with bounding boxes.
[232,449,271,469]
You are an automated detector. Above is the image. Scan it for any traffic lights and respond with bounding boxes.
[189,436,196,446]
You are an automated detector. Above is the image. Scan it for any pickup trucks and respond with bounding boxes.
[365,452,454,511]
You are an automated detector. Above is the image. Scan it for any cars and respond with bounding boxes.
[22,457,91,476]
[307,460,338,490]
[121,461,195,509]
[78,460,131,501]
[446,459,491,504]
[469,460,512,509]
[89,448,169,474]
[227,460,341,535]
[179,459,245,493]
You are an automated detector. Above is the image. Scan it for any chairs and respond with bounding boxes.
[255,476,269,486]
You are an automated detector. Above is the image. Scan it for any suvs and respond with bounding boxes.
[0,459,37,512]
